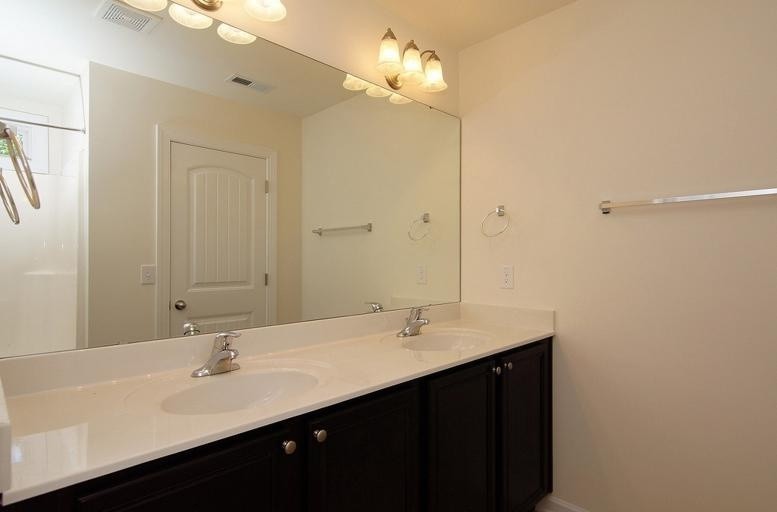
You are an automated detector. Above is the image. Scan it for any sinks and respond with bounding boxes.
[401,329,497,352]
[159,369,319,416]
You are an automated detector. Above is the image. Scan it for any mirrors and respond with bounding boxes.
[0,0,461,366]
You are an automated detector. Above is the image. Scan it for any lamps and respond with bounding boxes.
[246,0,307,27]
[383,25,450,97]
[337,73,413,110]
[121,3,254,48]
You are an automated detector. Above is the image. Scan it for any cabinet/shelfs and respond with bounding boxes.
[175,370,423,512]
[423,336,555,512]
[2,448,174,512]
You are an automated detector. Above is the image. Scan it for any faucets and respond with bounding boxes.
[183,322,201,337]
[363,302,385,312]
[396,307,431,338]
[191,332,242,378]
